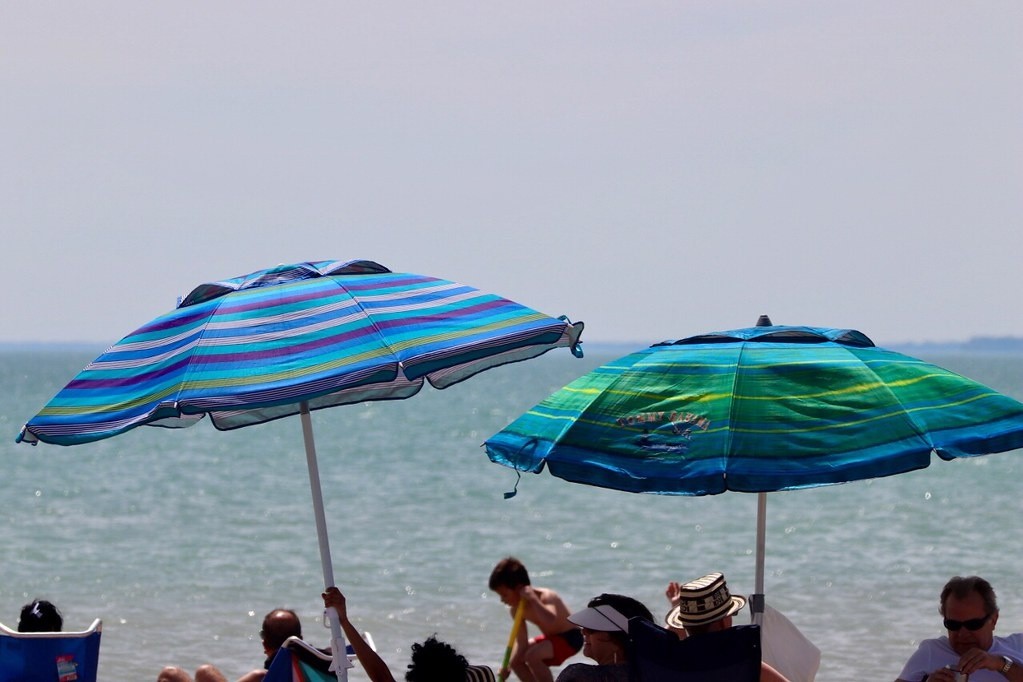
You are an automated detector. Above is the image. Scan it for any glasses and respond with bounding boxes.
[943,610,990,631]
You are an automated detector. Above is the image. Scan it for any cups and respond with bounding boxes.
[943,664,968,682]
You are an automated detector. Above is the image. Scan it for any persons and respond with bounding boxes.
[321,587,496,682]
[895,576,1023,682]
[18,599,63,632]
[556,573,789,682]
[158,609,366,682]
[489,557,583,682]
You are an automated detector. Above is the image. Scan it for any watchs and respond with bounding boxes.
[1002,655,1013,672]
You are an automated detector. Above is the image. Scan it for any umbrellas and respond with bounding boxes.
[15,259,584,682]
[482,314,1023,625]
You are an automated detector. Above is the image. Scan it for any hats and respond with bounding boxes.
[568,602,630,634]
[665,573,747,628]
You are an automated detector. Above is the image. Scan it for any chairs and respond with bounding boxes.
[0,618,101,682]
[625,616,762,682]
[261,632,376,682]
